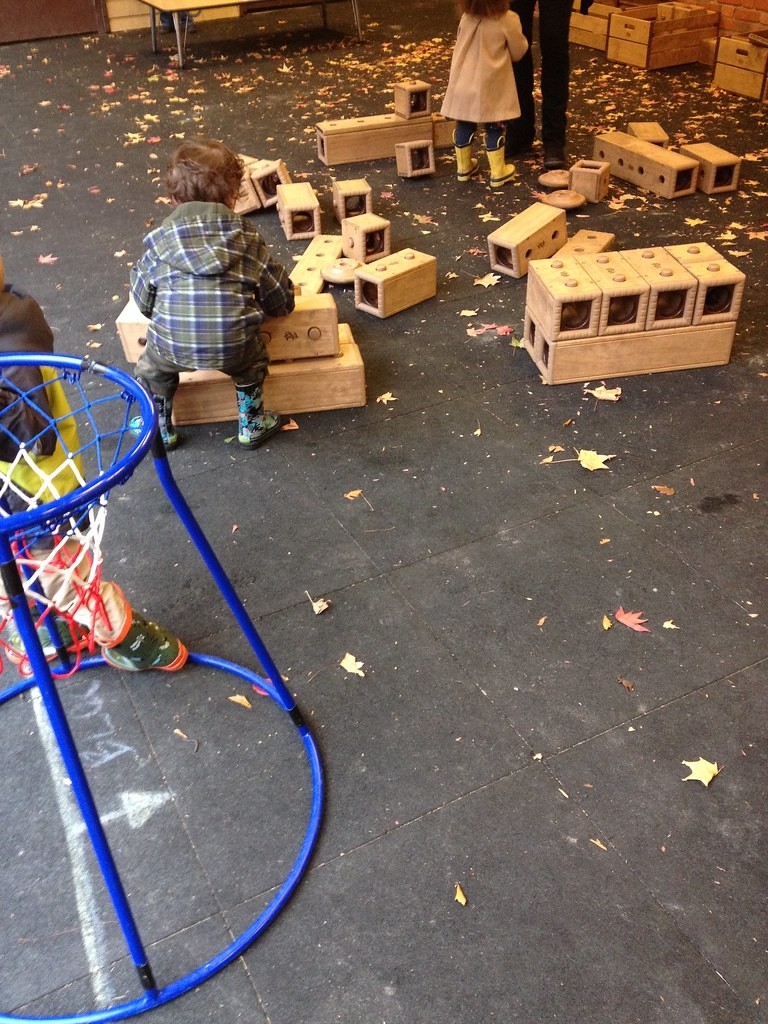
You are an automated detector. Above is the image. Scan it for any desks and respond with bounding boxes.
[138,0,365,70]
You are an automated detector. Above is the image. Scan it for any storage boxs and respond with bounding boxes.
[114,0,768,426]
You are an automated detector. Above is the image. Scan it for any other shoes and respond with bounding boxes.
[504,143,532,159]
[543,149,564,168]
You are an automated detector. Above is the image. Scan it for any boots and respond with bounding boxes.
[128,394,179,450]
[483,134,515,187]
[101,607,188,672]
[452,128,480,181]
[4,614,89,665]
[234,381,280,448]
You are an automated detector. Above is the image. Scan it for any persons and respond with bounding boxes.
[440,0,528,187]
[129,136,295,449]
[0,255,188,671]
[504,0,575,172]
